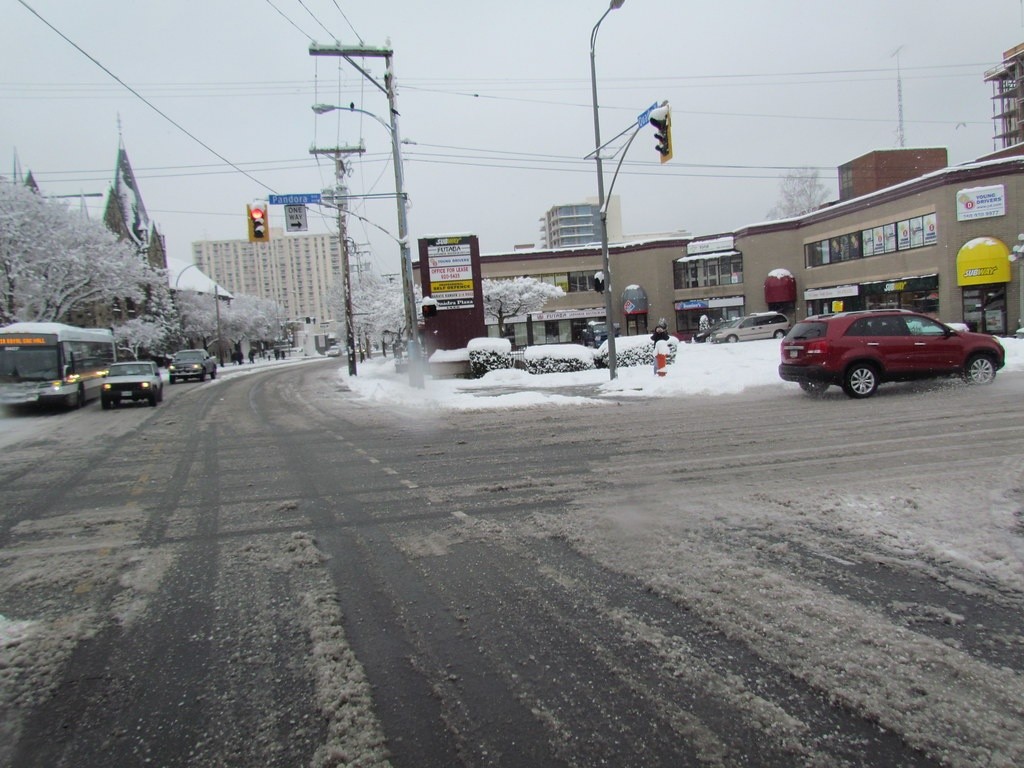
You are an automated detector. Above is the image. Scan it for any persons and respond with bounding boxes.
[248,349,255,364]
[274,347,280,360]
[231,351,243,365]
[650,322,669,374]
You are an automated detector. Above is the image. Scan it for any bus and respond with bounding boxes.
[0,322,119,414]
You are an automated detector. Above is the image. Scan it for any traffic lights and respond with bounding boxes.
[650,102,673,163]
[247,203,270,243]
[422,305,437,317]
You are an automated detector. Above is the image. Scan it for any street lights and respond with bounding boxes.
[310,103,412,339]
[589,0,625,382]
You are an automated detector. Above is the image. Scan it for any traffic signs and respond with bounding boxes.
[284,204,308,233]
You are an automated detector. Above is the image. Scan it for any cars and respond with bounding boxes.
[694,317,742,343]
[327,346,342,357]
[711,312,793,344]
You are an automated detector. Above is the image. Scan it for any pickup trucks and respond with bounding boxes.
[168,348,218,383]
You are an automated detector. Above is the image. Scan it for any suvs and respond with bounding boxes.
[100,361,164,410]
[778,307,1008,401]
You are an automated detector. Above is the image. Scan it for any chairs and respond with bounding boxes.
[870,319,883,336]
